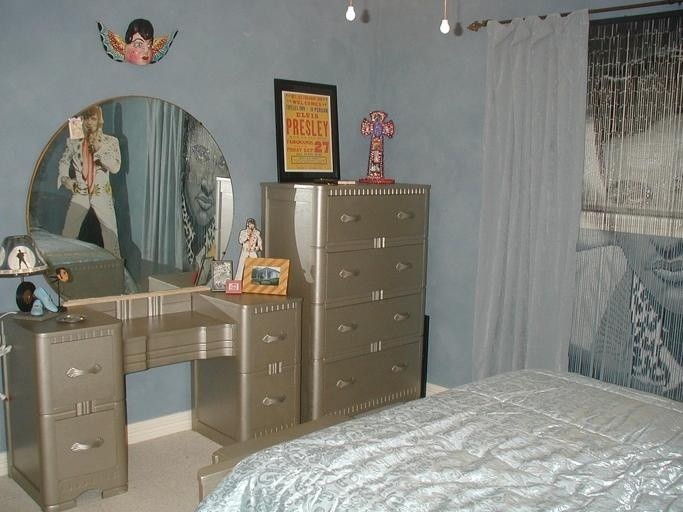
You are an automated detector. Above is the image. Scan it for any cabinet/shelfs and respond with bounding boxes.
[2,288,303,511]
[261,178,431,436]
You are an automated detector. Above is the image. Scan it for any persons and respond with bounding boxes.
[183,120,230,272]
[59,105,122,264]
[234,218,263,281]
[95,17,178,66]
[587,48,683,403]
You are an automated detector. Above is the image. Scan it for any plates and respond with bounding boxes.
[55,312,87,325]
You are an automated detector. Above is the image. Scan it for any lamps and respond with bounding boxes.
[0,235,51,311]
[361,0,370,22]
[345,1,357,22]
[454,0,464,35]
[440,0,452,34]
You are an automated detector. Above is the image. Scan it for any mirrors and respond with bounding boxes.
[27,93,237,309]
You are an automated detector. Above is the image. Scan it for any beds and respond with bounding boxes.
[30,227,143,298]
[199,368,683,507]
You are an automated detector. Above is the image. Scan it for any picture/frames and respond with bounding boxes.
[242,256,291,296]
[273,76,342,185]
[211,261,234,291]
[226,280,242,294]
